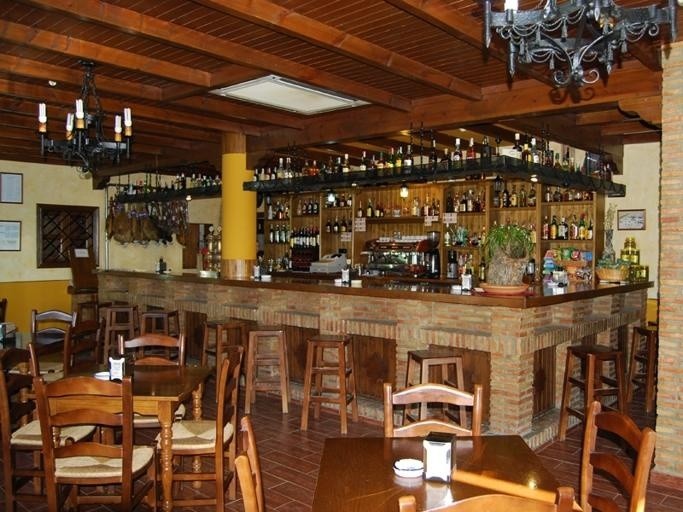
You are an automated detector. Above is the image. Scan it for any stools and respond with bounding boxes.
[139,309,180,360]
[555,319,659,444]
[300,335,359,435]
[244,326,291,415]
[401,349,467,430]
[103,304,139,366]
[77,300,111,357]
[201,318,248,404]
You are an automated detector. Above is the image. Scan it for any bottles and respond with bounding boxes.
[124,172,222,194]
[511,128,614,188]
[447,181,536,280]
[203,232,221,272]
[266,199,290,272]
[296,197,320,216]
[620,235,649,281]
[253,135,502,190]
[325,193,353,232]
[356,192,441,218]
[290,225,319,249]
[461,262,473,291]
[542,183,592,242]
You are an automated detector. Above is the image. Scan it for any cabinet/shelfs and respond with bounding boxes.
[483,0,678,91]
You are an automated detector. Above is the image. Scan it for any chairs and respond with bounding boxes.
[395,484,576,512]
[152,346,245,512]
[578,399,657,512]
[0,343,102,512]
[0,298,102,379]
[234,413,267,512]
[31,374,157,512]
[115,329,187,496]
[381,380,484,437]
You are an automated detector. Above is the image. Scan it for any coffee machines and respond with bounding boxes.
[362,237,431,278]
[423,230,441,279]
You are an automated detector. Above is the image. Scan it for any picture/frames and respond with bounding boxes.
[617,209,646,230]
[0,172,23,204]
[0,219,22,251]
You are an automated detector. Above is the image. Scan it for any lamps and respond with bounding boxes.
[35,56,133,175]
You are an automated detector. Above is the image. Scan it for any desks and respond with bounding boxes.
[311,434,582,512]
[48,356,213,512]
